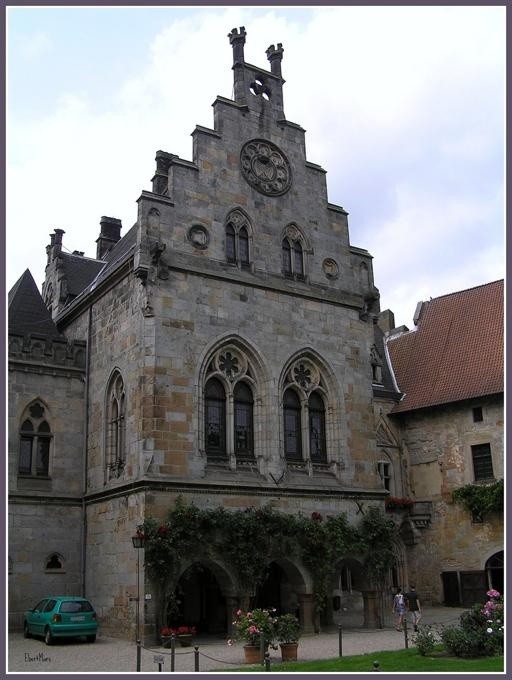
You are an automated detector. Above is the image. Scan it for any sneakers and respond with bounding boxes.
[395,624,419,632]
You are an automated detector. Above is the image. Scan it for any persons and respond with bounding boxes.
[390,586,408,633]
[403,583,424,633]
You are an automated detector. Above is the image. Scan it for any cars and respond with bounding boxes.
[23,597,98,645]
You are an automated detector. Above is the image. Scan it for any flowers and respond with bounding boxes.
[226,608,280,650]
[177,626,197,637]
[160,625,178,637]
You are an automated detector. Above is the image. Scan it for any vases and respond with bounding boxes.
[179,634,193,647]
[162,636,173,648]
[243,643,268,664]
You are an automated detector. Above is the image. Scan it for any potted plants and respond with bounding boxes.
[277,614,304,661]
